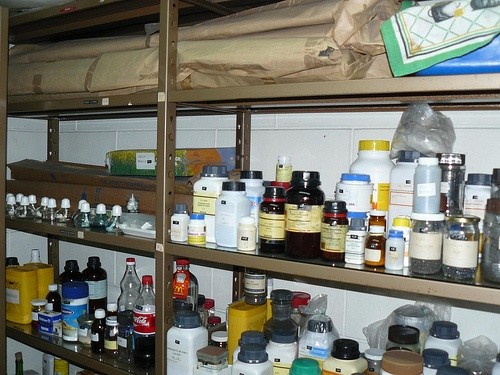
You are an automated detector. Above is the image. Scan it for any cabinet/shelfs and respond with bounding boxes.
[1,0,500,375]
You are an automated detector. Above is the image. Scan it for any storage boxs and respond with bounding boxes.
[105,147,236,177]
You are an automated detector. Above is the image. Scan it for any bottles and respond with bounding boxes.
[133,259,229,375]
[436,153,466,214]
[409,213,481,280]
[228,271,297,375]
[193,165,250,248]
[15,351,99,375]
[117,258,142,362]
[240,170,266,244]
[289,292,368,375]
[463,168,500,282]
[104,303,119,357]
[5,193,139,232]
[413,156,440,214]
[237,217,256,250]
[170,204,206,244]
[364,304,500,375]
[258,156,325,254]
[6,249,107,346]
[320,201,349,261]
[336,139,421,271]
